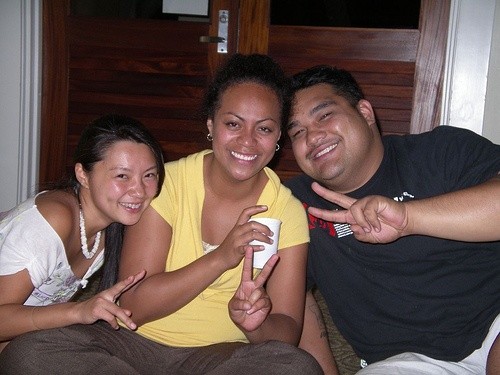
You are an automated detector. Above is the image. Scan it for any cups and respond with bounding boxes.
[248,216,282,270]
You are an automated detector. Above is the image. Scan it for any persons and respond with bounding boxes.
[1,56,327,375]
[0,111,168,353]
[281,59,500,375]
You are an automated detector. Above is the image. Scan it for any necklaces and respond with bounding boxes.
[77,197,104,260]
